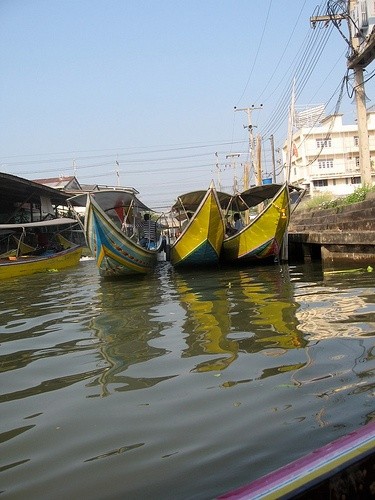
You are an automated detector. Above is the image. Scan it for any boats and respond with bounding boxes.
[221,178,307,269]
[66,184,166,277]
[0,171,82,281]
[164,178,234,272]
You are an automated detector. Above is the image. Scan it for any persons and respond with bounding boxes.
[136,213,169,248]
[175,212,192,238]
[226,213,242,237]
[23,228,48,256]
